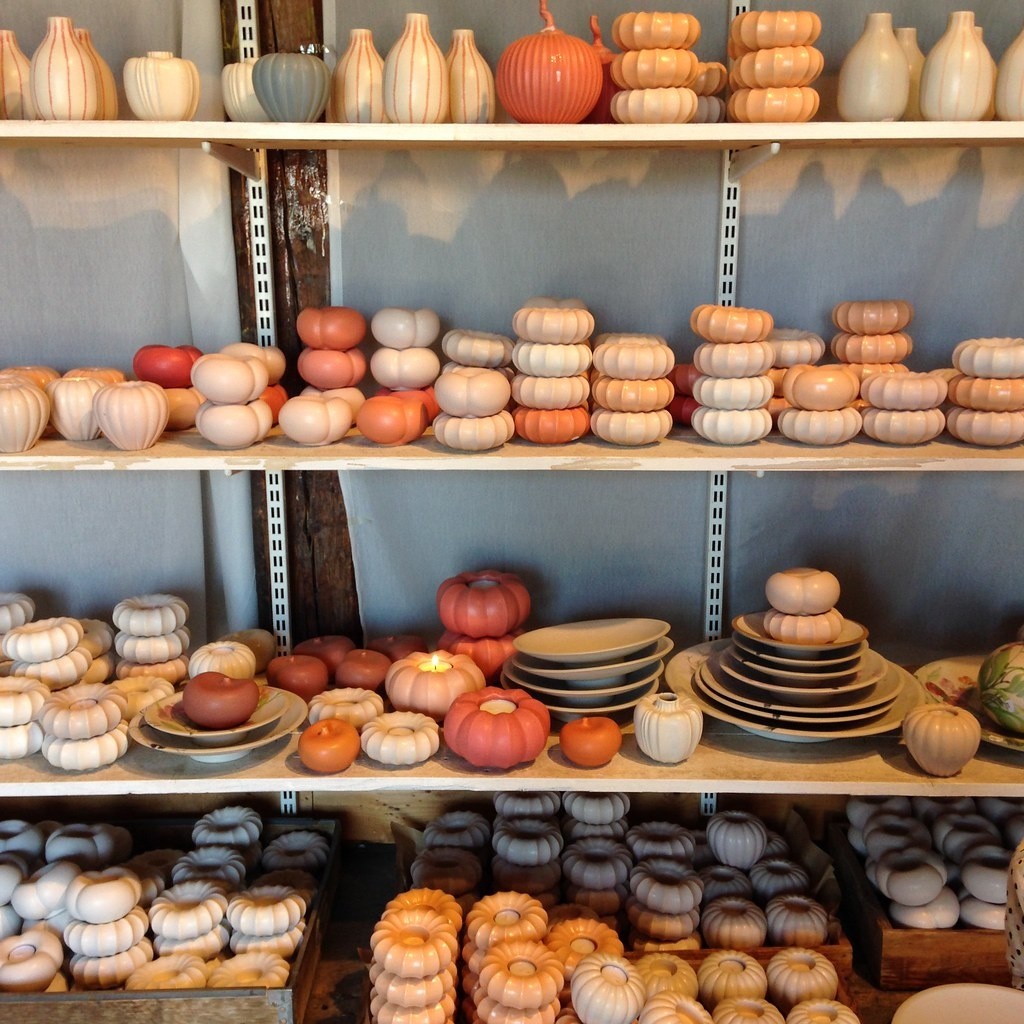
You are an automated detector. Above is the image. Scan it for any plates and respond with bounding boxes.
[919,655,1023,751]
[500,613,922,741]
[130,687,308,764]
[891,982,1023,1024]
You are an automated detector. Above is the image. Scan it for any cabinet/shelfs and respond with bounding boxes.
[1,118,1024,1024]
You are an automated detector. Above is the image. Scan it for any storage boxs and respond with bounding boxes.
[626,908,852,990]
[0,817,344,1024]
[822,820,1010,992]
